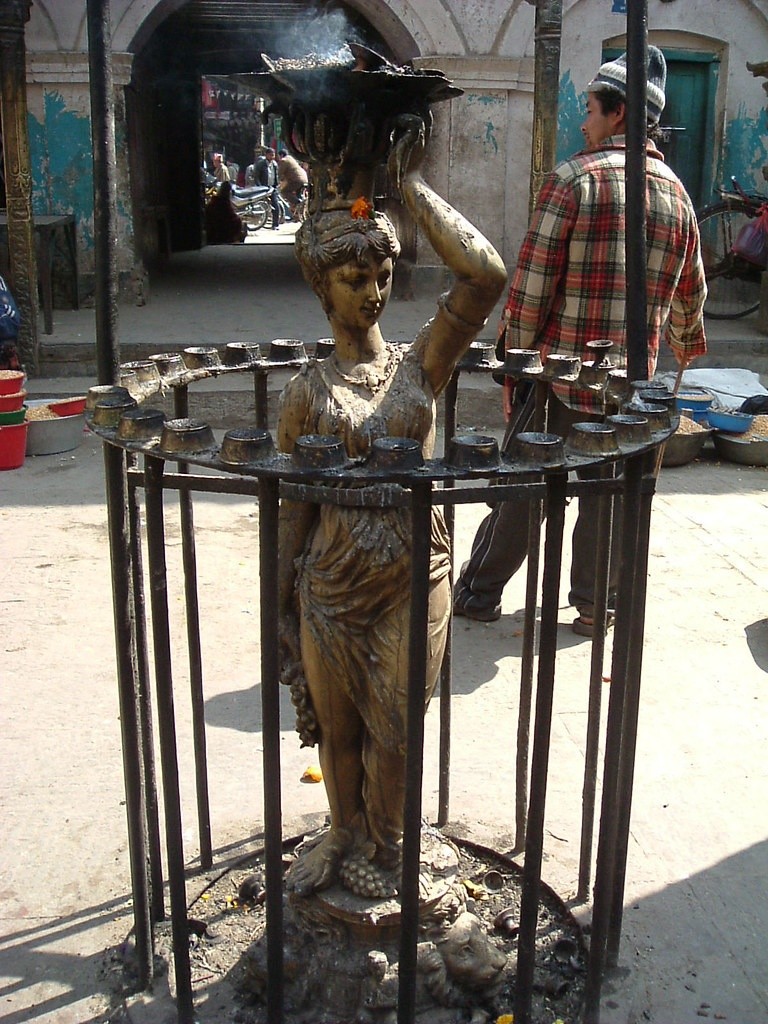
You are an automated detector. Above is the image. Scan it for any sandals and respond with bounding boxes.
[453,602,502,621]
[573,610,615,636]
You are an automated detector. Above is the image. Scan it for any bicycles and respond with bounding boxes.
[264,182,310,229]
[695,166,768,320]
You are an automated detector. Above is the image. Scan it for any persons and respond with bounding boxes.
[203,148,308,245]
[276,108,505,900]
[451,44,708,637]
[0,274,21,370]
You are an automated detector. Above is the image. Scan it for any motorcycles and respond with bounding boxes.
[205,174,274,231]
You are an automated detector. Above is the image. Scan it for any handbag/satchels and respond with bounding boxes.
[733,204,768,267]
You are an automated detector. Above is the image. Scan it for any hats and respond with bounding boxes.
[586,44,667,123]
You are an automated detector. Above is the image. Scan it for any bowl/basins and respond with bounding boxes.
[444,340,678,473]
[86,384,277,465]
[294,434,348,472]
[0,370,24,395]
[662,422,714,466]
[708,414,768,466]
[705,408,753,432]
[0,389,26,412]
[48,399,86,416]
[372,437,425,472]
[0,404,28,425]
[118,337,335,392]
[24,399,86,455]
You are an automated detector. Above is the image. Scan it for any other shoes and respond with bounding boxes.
[272,226,279,230]
[292,198,302,207]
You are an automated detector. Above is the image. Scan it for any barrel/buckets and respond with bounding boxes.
[674,391,715,425]
[0,419,29,471]
